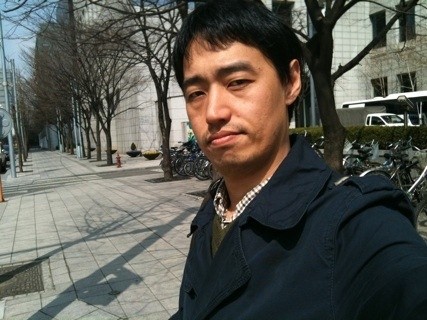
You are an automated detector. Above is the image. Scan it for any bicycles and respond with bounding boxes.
[160,137,427,246]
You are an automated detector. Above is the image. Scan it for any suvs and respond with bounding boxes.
[397,115,420,127]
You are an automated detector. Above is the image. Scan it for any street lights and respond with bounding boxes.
[42,42,82,158]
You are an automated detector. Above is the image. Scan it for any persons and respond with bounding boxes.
[162,0,427,320]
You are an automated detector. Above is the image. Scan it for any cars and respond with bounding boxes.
[0,142,7,168]
[365,113,405,127]
